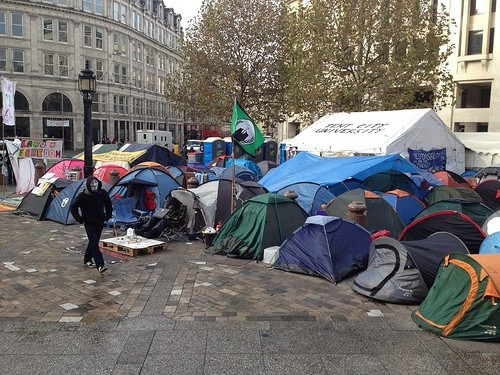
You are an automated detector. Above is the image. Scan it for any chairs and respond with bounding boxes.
[115,208,188,242]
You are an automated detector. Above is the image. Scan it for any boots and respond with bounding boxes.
[135,227,148,235]
[144,229,159,239]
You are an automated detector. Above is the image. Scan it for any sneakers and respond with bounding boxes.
[83,259,96,267]
[99,265,107,273]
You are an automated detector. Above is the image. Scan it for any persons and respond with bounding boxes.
[164,142,196,161]
[94,137,129,145]
[70,176,112,274]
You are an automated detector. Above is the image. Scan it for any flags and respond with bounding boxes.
[231,99,265,159]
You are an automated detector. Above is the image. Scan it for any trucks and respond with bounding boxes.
[136,129,173,153]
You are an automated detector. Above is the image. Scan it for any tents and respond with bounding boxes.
[452,131,500,173]
[12,144,500,343]
[287,108,465,176]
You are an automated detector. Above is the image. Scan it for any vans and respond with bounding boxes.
[185,140,204,152]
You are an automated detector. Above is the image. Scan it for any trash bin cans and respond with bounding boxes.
[187,152,202,163]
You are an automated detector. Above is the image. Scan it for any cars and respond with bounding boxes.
[0,140,8,160]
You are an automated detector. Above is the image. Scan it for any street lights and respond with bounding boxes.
[76,59,96,178]
